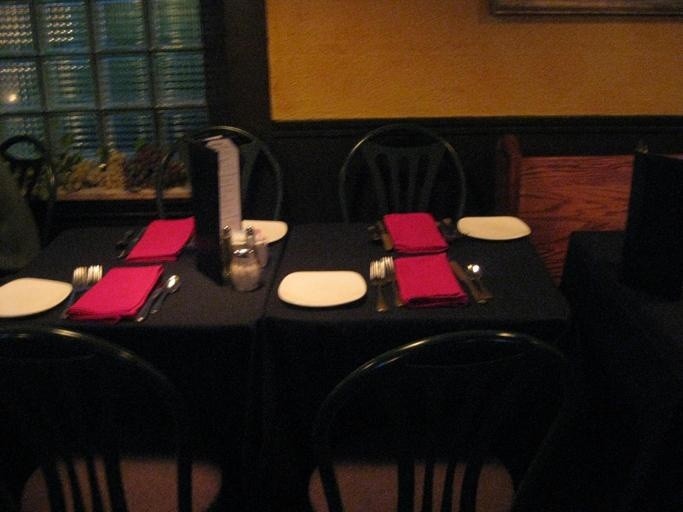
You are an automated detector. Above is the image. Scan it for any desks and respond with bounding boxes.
[258,222,575,494]
[0,218,290,489]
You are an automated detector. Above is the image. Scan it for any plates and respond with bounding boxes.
[0,277,73,320]
[277,270,367,308]
[457,216,532,241]
[228,220,288,246]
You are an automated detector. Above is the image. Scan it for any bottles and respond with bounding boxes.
[219,225,269,292]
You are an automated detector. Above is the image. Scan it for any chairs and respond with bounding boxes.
[314,327,567,508]
[337,122,467,223]
[155,122,286,218]
[5,324,195,508]
[1,134,56,285]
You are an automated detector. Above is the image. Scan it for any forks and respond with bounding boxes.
[59,263,104,320]
[370,256,404,313]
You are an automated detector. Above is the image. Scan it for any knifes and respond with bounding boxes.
[376,219,393,251]
[136,276,168,323]
[448,259,486,306]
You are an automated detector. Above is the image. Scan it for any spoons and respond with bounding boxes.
[467,263,494,299]
[149,275,181,315]
